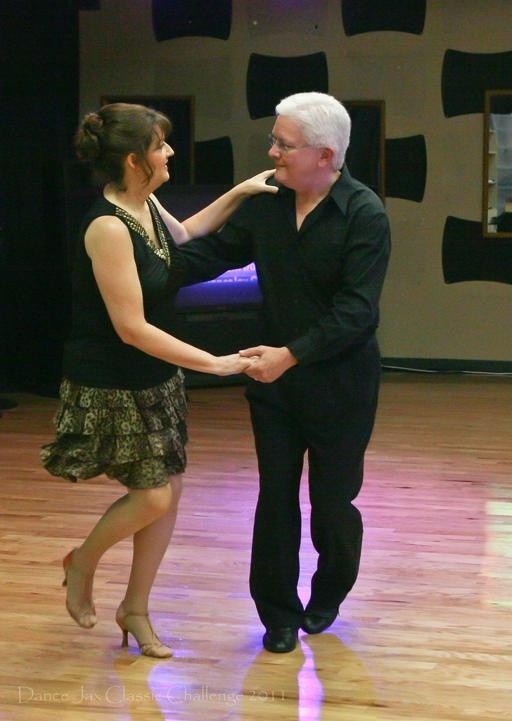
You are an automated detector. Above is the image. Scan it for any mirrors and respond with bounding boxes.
[482,89,512,238]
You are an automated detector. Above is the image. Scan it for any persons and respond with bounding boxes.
[39,102,281,657]
[178,89,393,652]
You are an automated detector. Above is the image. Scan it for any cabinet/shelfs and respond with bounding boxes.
[487,112,498,234]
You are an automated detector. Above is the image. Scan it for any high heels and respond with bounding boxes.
[63,548,96,628]
[116,602,172,658]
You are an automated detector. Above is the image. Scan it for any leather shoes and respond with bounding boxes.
[263,606,304,653]
[301,572,338,633]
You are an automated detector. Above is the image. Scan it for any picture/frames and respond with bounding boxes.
[338,99,386,210]
[100,94,196,190]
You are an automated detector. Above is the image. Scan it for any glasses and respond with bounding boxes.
[266,132,313,156]
